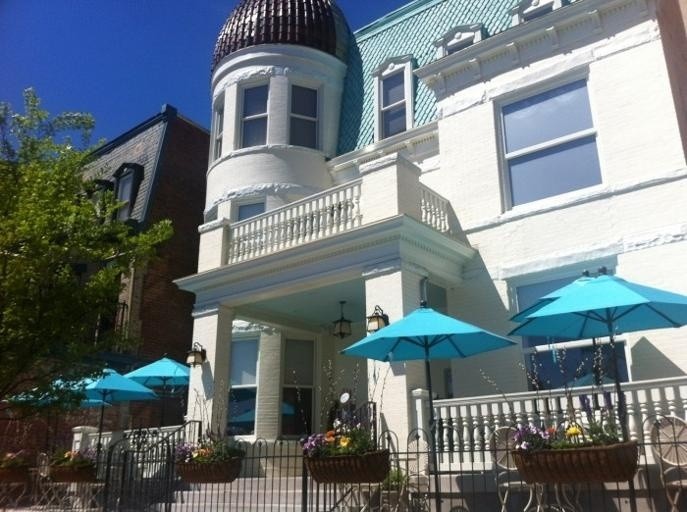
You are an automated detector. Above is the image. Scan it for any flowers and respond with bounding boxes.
[303,424,378,454]
[50,450,96,468]
[511,420,621,450]
[171,435,243,463]
[0,452,22,468]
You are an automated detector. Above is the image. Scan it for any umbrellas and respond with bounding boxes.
[120,351,191,426]
[48,366,160,452]
[506,265,687,512]
[504,268,604,384]
[338,299,520,512]
[227,397,296,426]
[0,373,112,452]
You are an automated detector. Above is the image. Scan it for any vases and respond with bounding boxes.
[307,448,390,482]
[0,466,27,483]
[178,462,240,483]
[40,464,97,483]
[510,439,639,485]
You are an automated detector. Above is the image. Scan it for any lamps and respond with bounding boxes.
[331,301,353,343]
[366,303,389,333]
[187,340,207,369]
